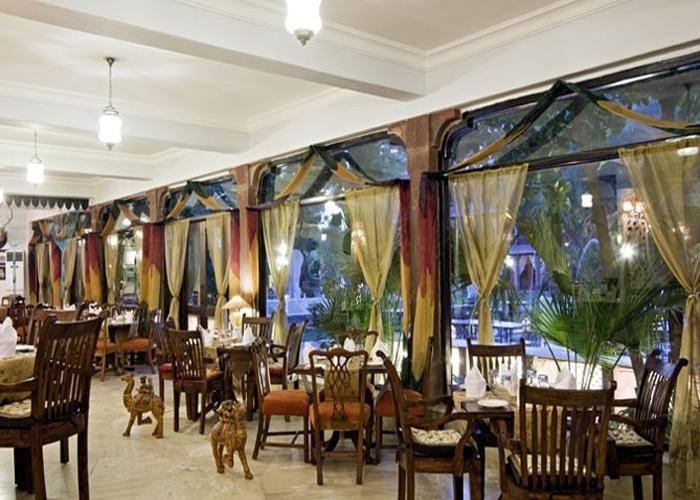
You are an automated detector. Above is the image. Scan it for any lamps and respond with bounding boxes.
[285,1,323,46]
[26,129,46,188]
[96,58,123,150]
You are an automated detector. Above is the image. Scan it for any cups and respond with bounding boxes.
[538,375,548,388]
[526,369,536,386]
[502,374,512,394]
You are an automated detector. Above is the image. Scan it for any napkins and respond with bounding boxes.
[497,365,516,391]
[463,367,485,397]
[556,369,576,392]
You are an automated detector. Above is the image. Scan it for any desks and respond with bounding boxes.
[292,360,388,464]
[452,387,562,455]
[201,335,269,414]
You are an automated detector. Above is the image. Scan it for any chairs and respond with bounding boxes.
[2,295,260,399]
[1,315,100,500]
[496,379,617,498]
[250,340,311,464]
[308,349,371,488]
[165,329,230,433]
[240,312,277,340]
[376,350,483,499]
[259,320,309,423]
[375,336,437,465]
[468,338,526,391]
[567,350,686,496]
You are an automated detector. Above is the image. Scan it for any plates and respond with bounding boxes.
[477,399,509,409]
[361,362,383,367]
